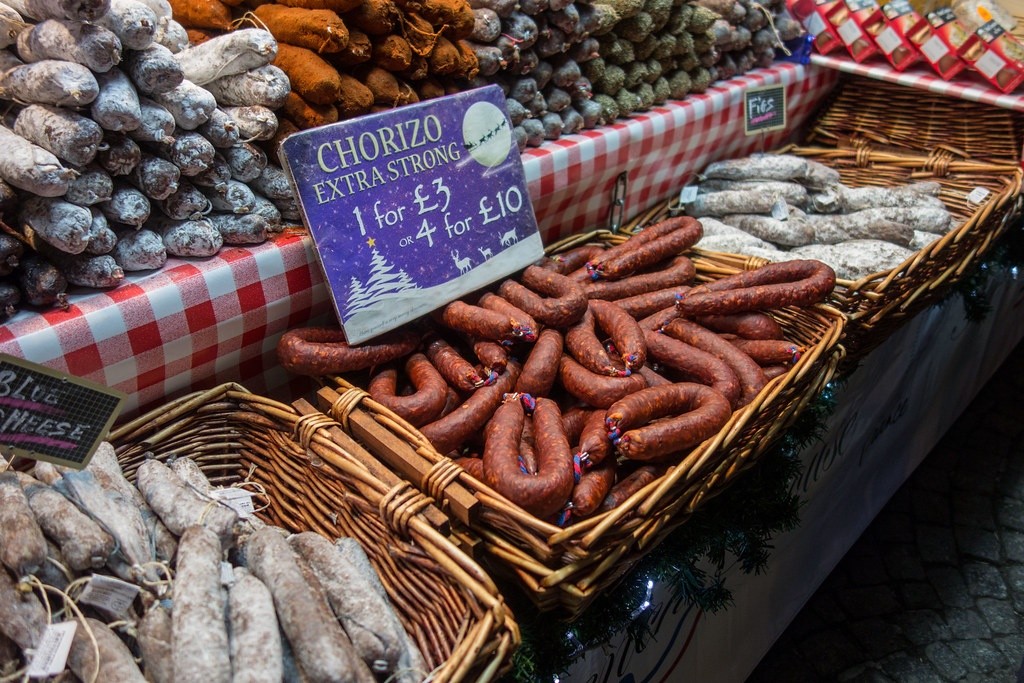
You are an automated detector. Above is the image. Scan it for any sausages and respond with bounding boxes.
[278,214,837,526]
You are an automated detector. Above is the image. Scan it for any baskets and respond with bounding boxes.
[315,229,848,625]
[618,142,1024,339]
[803,73,1024,175]
[15,380,524,683]
